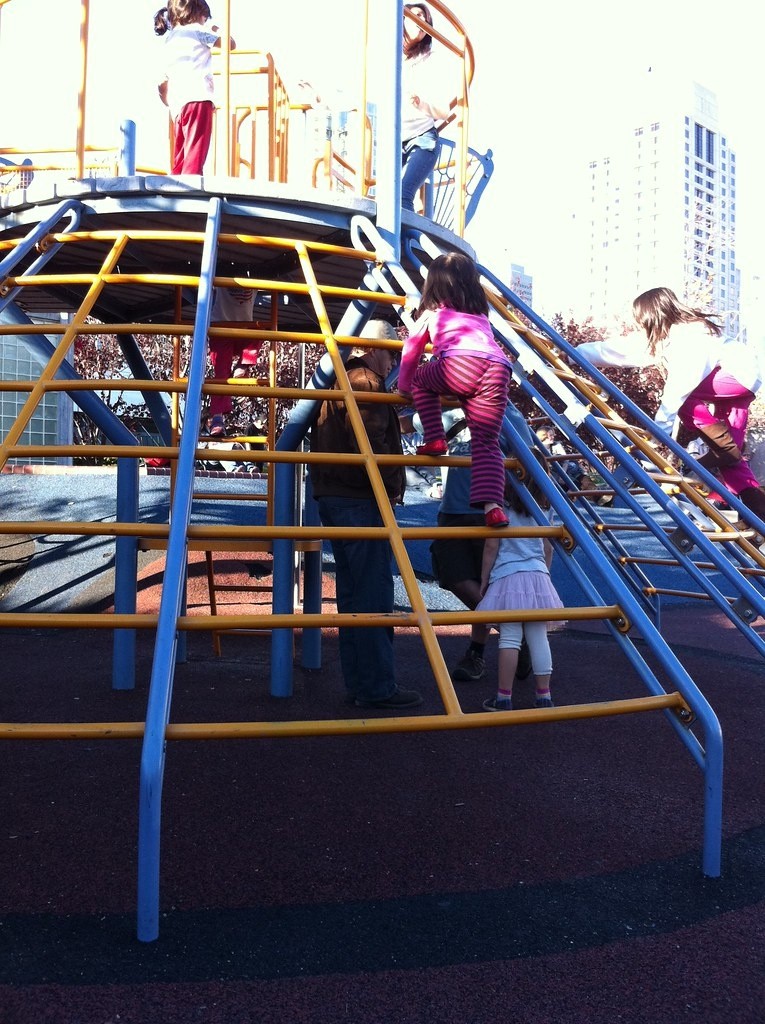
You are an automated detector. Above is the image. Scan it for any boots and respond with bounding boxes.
[682,422,743,471]
[730,486,765,530]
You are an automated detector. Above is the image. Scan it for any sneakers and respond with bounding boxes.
[485,509,511,527]
[209,416,227,437]
[529,700,555,709]
[415,439,448,456]
[482,697,513,712]
[515,639,533,680]
[233,363,250,379]
[234,465,246,472]
[249,466,260,473]
[452,648,488,681]
[344,684,424,709]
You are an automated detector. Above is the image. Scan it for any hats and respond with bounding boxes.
[359,318,400,340]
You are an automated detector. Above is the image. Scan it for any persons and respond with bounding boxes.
[206,268,264,437]
[194,406,261,476]
[311,319,424,708]
[556,288,765,532]
[530,422,616,507]
[245,415,268,474]
[399,395,537,683]
[390,250,516,528]
[474,447,568,713]
[153,0,236,175]
[400,3,451,216]
